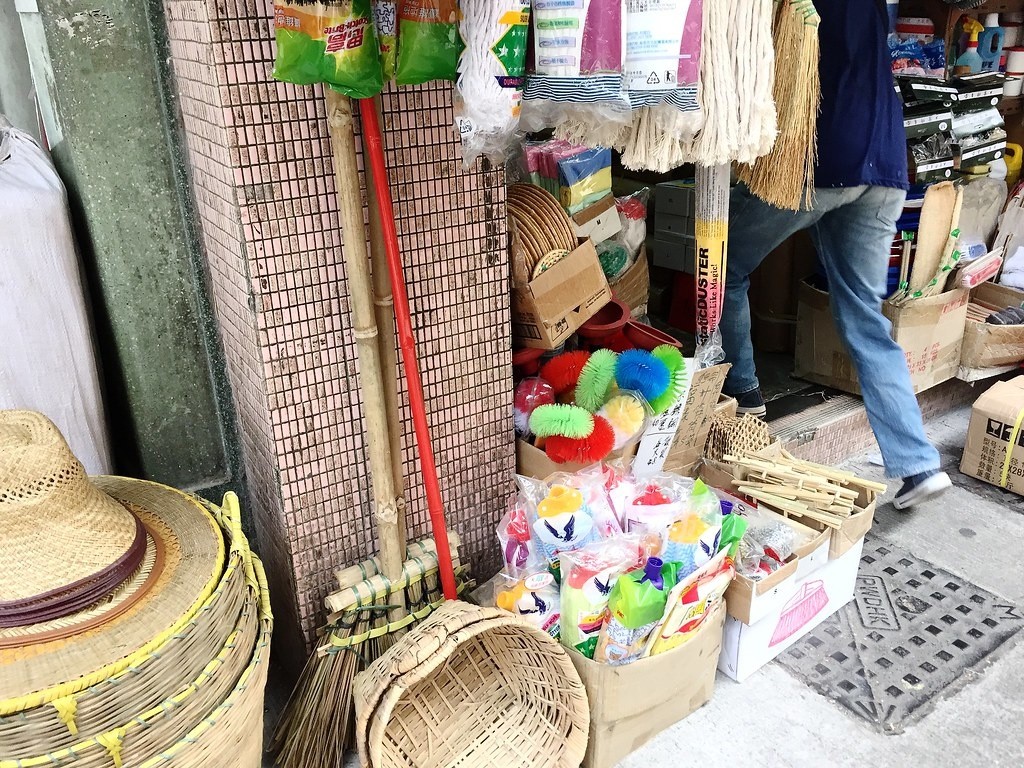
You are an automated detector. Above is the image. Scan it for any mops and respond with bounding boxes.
[454,0,780,174]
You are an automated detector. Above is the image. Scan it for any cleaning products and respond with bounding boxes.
[955,12,1005,74]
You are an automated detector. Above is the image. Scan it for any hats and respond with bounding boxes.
[1,409,226,709]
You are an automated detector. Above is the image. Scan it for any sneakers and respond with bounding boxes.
[732,387,767,419]
[892,469,953,509]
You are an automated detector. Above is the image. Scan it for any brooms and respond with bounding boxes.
[722,0,823,215]
[265,85,481,768]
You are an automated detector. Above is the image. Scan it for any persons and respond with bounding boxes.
[716,1,956,514]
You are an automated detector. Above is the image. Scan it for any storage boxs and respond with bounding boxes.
[960,297,1024,369]
[897,68,1008,184]
[565,597,727,768]
[956,375,1024,496]
[795,526,832,581]
[717,393,739,416]
[726,554,800,627]
[976,280,1024,309]
[684,247,694,275]
[710,433,781,475]
[655,177,690,217]
[570,191,622,246]
[700,465,878,558]
[686,218,695,247]
[653,212,688,246]
[508,235,613,350]
[716,534,865,684]
[690,189,695,219]
[515,437,638,479]
[791,279,971,396]
[653,239,686,272]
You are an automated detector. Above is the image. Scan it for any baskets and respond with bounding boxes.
[351,599,590,766]
[2,489,273,767]
